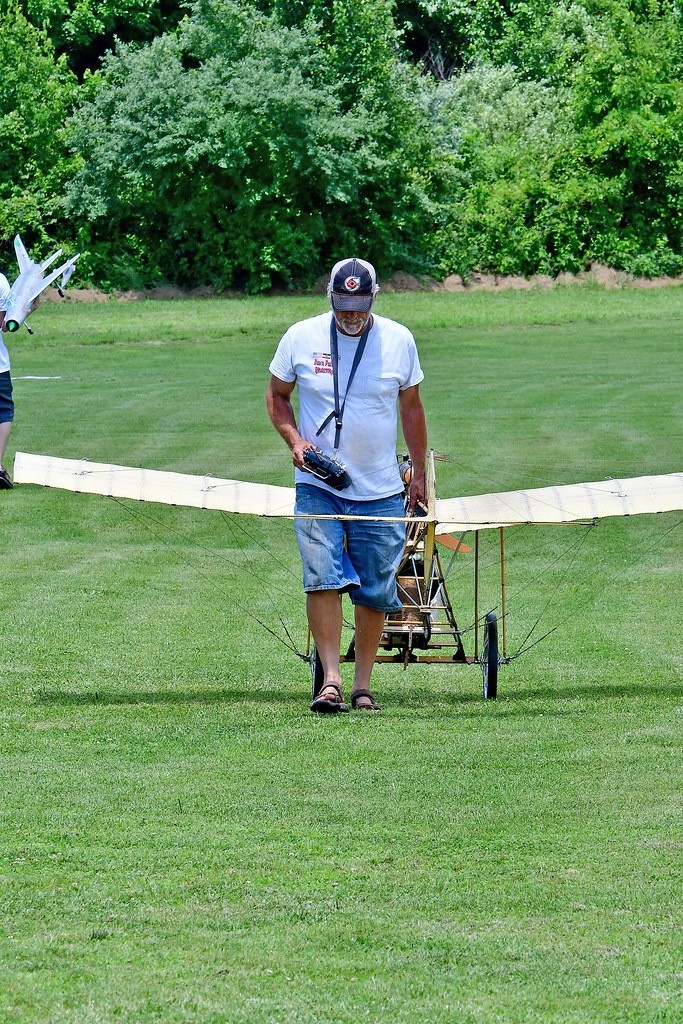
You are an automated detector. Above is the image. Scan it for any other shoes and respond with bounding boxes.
[0,471,14,488]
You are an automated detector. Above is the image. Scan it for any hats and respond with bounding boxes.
[329,258,381,312]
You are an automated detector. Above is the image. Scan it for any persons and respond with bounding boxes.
[265,257,429,714]
[0,273,39,489]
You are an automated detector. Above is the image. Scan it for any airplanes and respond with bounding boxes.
[11,449,683,701]
[2,234,80,334]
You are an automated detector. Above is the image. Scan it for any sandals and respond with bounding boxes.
[309,682,350,712]
[352,689,380,712]
